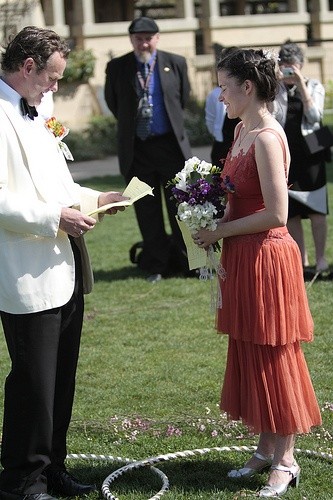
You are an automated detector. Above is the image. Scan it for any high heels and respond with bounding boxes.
[228,451,275,480]
[256,461,300,498]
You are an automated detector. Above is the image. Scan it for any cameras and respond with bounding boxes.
[281,68,294,77]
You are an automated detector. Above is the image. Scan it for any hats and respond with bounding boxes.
[128,17,160,34]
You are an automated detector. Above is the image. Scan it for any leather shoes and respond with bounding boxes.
[45,470,92,496]
[0,493,59,500]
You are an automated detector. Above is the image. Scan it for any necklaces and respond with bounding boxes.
[238,111,269,146]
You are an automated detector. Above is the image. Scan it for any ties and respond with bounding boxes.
[137,63,152,143]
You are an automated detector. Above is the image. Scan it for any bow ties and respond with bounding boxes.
[20,97,39,120]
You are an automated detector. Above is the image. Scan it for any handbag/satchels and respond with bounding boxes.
[302,108,333,154]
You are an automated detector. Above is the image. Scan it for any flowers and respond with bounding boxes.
[163,157,240,258]
[44,116,68,143]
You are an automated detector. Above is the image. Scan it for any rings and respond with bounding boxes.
[197,239,202,245]
[78,229,83,236]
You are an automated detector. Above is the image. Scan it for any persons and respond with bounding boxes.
[0,25,131,500]
[191,48,322,499]
[205,46,240,219]
[104,17,198,279]
[269,42,332,281]
